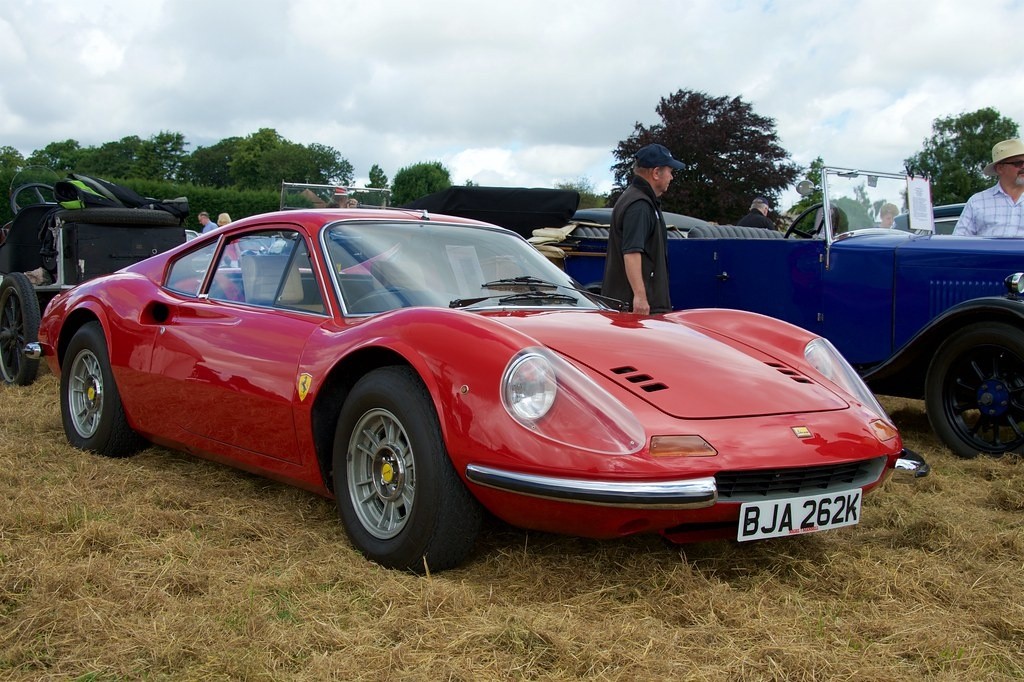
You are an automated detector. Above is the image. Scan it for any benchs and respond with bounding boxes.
[570,224,792,240]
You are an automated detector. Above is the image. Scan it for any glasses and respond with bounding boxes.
[999,160,1024,167]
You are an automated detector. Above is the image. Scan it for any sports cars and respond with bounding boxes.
[23,208,930,576]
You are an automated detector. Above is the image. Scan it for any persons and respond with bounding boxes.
[951,138,1024,237]
[217,213,241,268]
[601,144,686,316]
[736,197,776,230]
[327,195,357,208]
[198,212,219,253]
[874,203,899,228]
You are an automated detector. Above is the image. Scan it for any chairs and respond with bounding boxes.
[239,253,327,314]
[371,260,426,315]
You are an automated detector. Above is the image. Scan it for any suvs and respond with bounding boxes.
[534,167,1024,461]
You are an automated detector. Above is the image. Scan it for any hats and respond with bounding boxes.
[984,139,1024,176]
[635,143,686,169]
[751,196,768,204]
